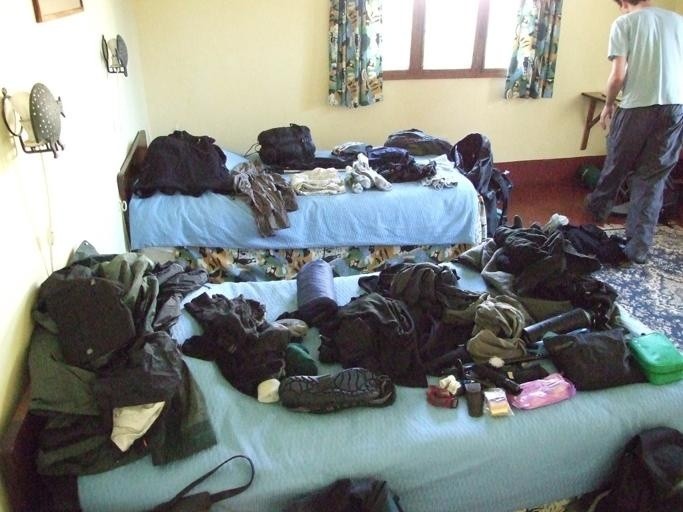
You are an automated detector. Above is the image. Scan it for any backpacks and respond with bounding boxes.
[385,128,453,157]
[446,133,515,237]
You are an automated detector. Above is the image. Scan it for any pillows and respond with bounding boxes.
[296,259,337,322]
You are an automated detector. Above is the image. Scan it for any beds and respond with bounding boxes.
[0,260,683,512]
[118,130,487,276]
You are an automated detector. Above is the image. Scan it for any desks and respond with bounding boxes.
[581,91,621,148]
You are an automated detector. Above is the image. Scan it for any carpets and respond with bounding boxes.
[584,222,683,365]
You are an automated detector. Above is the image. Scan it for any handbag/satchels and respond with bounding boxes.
[605,426,683,512]
[244,123,315,165]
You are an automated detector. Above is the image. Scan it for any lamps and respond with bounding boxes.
[1,84,66,158]
[102,33,128,77]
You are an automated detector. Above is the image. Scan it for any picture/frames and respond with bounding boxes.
[33,0,84,22]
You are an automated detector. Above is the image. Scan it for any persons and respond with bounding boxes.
[585,1,682,262]
